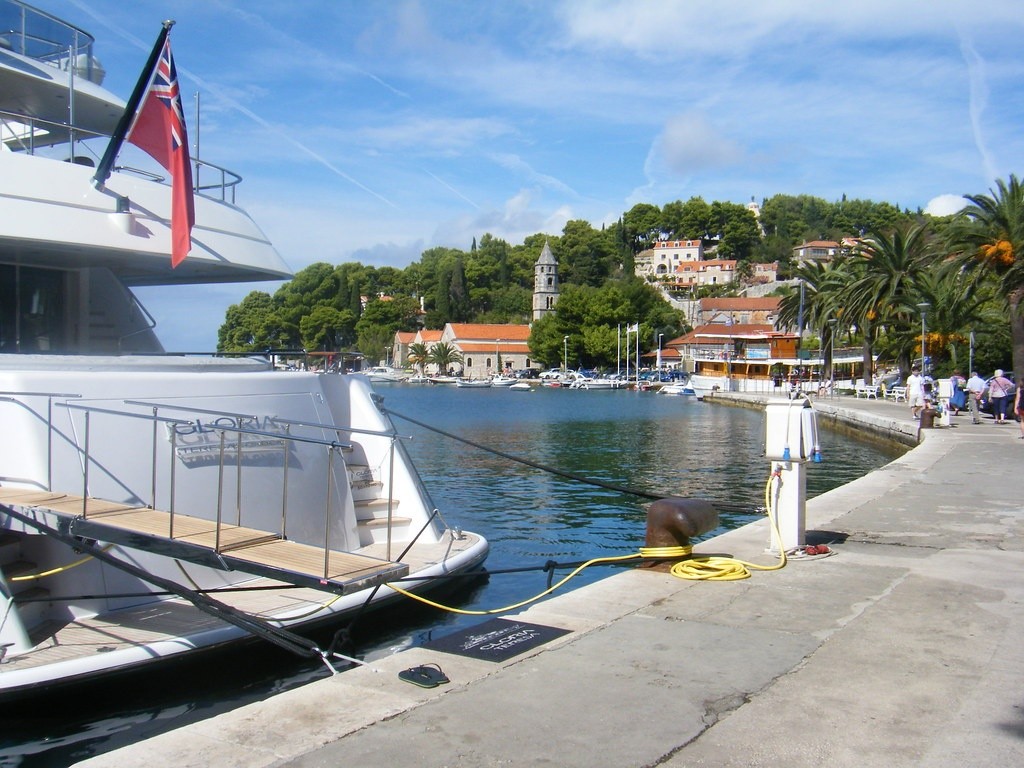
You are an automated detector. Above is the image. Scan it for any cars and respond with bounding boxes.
[953,370,1015,412]
[516,368,689,382]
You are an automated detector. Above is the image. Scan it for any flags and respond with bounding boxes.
[126,37,195,269]
[628,324,638,332]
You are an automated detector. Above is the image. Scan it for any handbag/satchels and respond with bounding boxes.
[957,379,966,388]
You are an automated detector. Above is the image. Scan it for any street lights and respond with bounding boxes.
[496,339,500,370]
[384,346,391,366]
[827,318,837,398]
[915,302,930,378]
[564,335,569,372]
[658,333,664,381]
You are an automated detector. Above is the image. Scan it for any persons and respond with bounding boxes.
[720,350,730,360]
[667,365,672,373]
[966,371,985,424]
[988,369,1015,424]
[825,377,831,389]
[504,366,512,377]
[593,366,597,371]
[949,369,966,415]
[906,366,925,419]
[1014,378,1024,439]
[707,351,715,359]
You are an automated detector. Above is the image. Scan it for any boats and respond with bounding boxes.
[510,383,531,391]
[489,371,517,385]
[632,380,695,395]
[540,372,628,389]
[0,2,488,753]
[346,366,462,384]
[456,378,493,389]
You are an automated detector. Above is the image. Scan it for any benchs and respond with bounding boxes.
[855,386,879,398]
[884,385,907,404]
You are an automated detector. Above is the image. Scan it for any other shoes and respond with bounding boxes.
[1000,420,1005,424]
[913,416,919,419]
[1018,436,1024,439]
[994,420,999,424]
[955,408,958,415]
